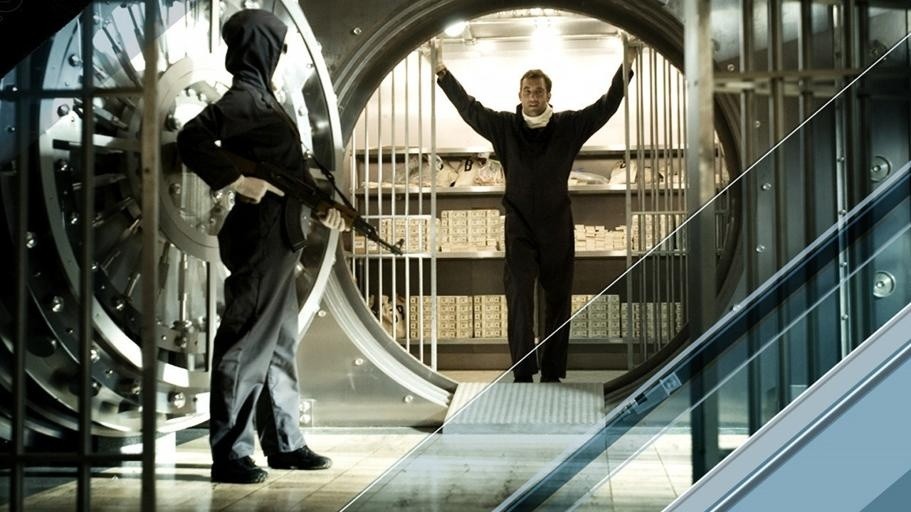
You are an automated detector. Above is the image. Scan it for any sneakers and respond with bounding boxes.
[268,445,333,470]
[211,455,267,483]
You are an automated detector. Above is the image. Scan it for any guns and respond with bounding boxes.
[224,150,406,256]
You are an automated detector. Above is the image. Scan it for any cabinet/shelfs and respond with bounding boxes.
[350,144,728,345]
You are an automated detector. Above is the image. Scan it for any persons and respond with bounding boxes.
[174,6,347,487]
[417,27,642,384]
